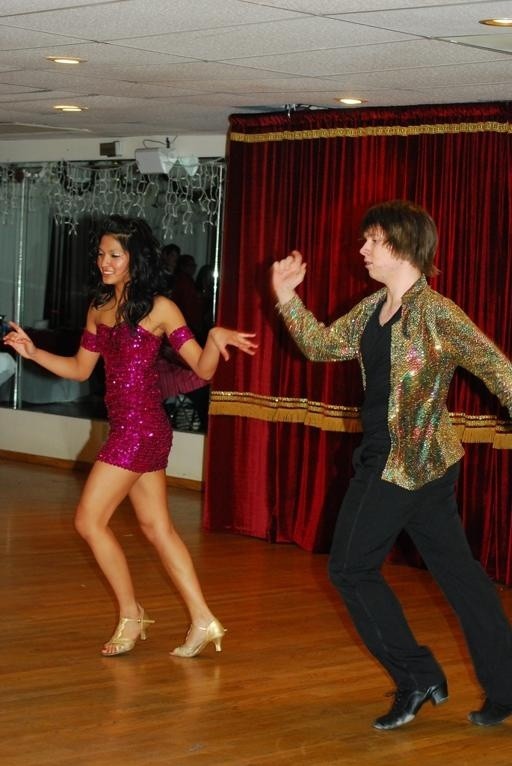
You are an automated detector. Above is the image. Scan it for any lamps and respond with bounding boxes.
[136,149,175,176]
[168,158,199,178]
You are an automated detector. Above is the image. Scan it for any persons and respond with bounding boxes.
[3,214,258,658]
[271,201,512,731]
[147,243,220,349]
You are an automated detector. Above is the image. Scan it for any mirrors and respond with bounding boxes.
[0,158,226,435]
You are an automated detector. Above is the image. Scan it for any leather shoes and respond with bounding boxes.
[466,697,512,726]
[373,678,448,730]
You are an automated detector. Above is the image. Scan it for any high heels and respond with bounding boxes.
[101,602,155,656]
[169,618,225,658]
